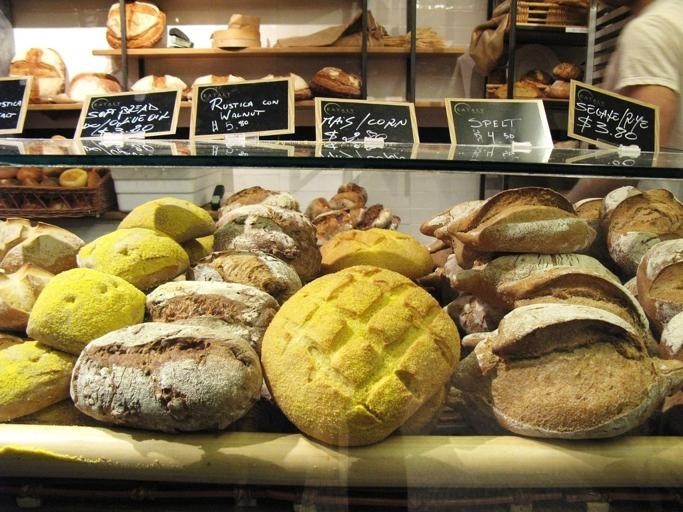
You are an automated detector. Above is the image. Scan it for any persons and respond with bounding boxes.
[561,1,683,203]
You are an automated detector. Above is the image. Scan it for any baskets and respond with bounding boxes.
[516,0,588,26]
[0,170,112,219]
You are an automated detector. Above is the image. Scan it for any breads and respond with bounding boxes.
[0,167,683,449]
[8,0,363,103]
[494,63,584,98]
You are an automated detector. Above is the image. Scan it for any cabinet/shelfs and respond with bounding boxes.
[475,13,592,149]
[87,26,461,146]
[0,131,682,511]
